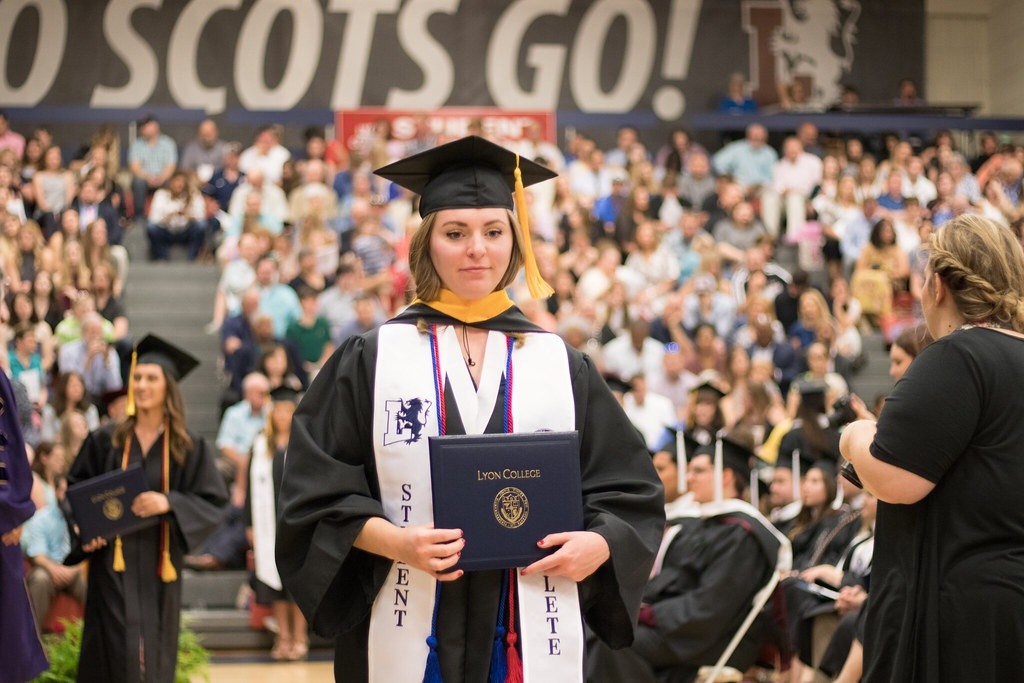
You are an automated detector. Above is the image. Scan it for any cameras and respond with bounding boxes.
[828,395,857,430]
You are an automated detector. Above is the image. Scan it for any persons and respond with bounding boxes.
[0,72,1023,683]
[275,136,666,683]
[839,213,1024,683]
[62,333,227,683]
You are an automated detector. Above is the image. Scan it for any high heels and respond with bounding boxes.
[286,641,309,661]
[272,640,287,661]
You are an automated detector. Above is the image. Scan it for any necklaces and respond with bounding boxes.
[955,320,1002,333]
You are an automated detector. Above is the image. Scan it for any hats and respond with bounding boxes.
[373,136,559,302]
[265,384,299,437]
[653,382,888,523]
[118,333,200,416]
